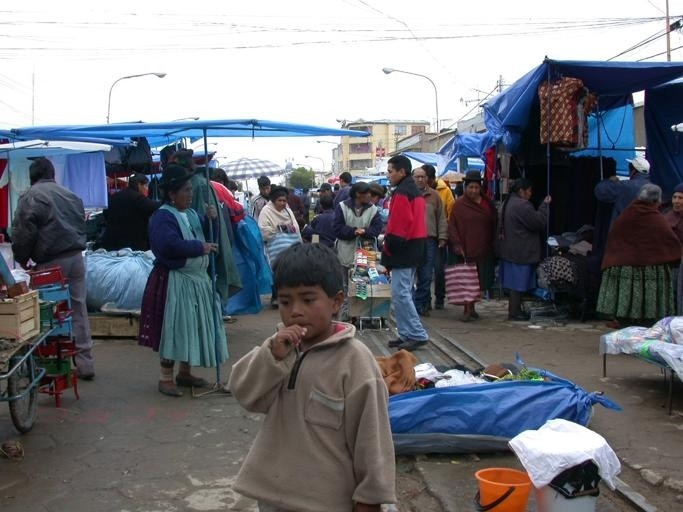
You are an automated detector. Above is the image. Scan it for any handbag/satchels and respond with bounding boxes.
[444,263,480,305]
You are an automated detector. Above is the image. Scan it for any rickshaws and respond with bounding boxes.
[0,269,74,434]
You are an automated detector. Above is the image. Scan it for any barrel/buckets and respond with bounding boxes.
[473,468,532,512]
[536,480,597,512]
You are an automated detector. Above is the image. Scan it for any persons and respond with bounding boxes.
[227,241,397,512]
[306,156,551,350]
[93,157,311,396]
[594,157,683,328]
[11,159,94,381]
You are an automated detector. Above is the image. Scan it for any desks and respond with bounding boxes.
[602,329,678,414]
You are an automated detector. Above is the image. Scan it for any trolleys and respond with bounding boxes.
[345,232,390,331]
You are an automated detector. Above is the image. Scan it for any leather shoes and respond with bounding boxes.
[176,376,203,387]
[158,381,183,396]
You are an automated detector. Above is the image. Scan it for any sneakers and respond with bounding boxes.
[388,339,402,348]
[398,339,427,352]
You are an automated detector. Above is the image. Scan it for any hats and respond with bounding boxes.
[368,183,385,198]
[626,157,649,175]
[462,171,485,182]
[316,183,331,191]
[158,163,197,190]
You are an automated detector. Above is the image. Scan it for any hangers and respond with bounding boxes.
[547,63,563,82]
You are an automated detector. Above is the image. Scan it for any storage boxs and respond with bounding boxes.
[0,288,41,344]
[347,270,392,319]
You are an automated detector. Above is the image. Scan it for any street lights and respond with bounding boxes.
[105,70,167,126]
[379,65,441,151]
[160,114,376,189]
